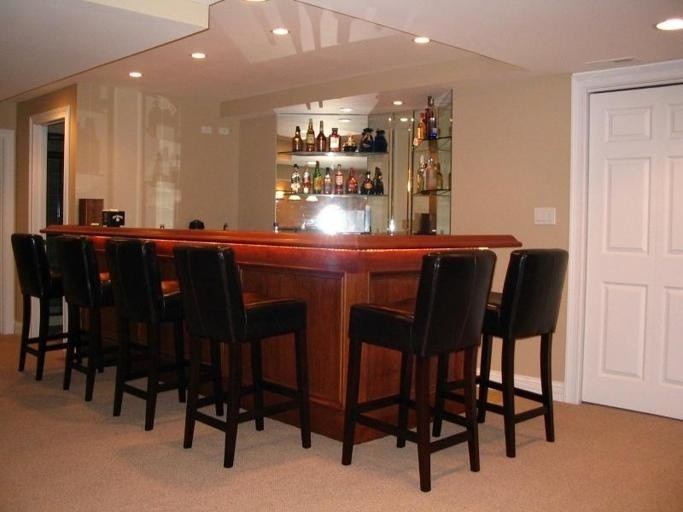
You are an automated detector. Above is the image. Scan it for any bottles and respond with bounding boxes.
[414,96,442,192]
[413,212,435,235]
[290,117,387,195]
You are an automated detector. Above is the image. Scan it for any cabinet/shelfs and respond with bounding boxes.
[275,103,451,234]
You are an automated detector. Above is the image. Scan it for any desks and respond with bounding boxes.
[40,225,523,447]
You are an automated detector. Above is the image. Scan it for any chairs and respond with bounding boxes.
[51,235,114,402]
[170,246,312,468]
[10,233,83,382]
[341,249,498,491]
[433,247,569,458]
[103,237,224,432]
